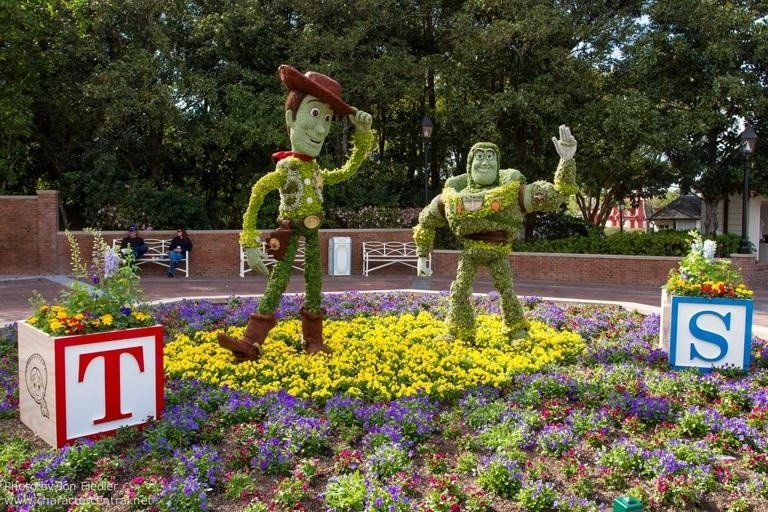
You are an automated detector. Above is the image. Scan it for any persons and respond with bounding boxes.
[168,227,193,277]
[120,227,149,264]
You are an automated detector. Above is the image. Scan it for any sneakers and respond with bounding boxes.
[168,273,173,278]
[121,249,126,256]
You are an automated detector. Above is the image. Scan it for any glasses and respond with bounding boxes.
[129,230,134,232]
[177,231,181,232]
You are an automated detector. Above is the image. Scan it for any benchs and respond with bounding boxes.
[239,239,309,278]
[361,240,434,277]
[114,238,191,278]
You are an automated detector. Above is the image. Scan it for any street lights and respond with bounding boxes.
[420,112,434,206]
[617,197,627,235]
[736,121,758,255]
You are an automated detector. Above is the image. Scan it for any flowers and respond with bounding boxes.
[22,225,157,337]
[659,231,753,300]
